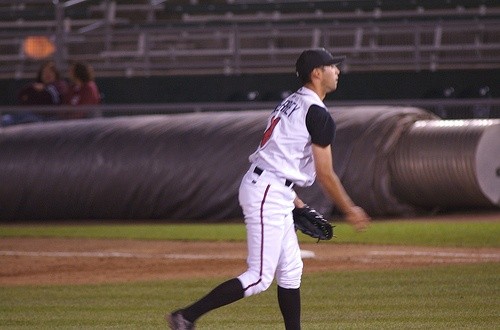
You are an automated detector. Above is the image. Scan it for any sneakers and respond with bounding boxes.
[170,310,194,330]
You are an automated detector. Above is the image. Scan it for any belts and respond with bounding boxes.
[253,166,296,188]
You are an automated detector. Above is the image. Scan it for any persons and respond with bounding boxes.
[166,48,372,330]
[20,61,68,107]
[62,59,101,120]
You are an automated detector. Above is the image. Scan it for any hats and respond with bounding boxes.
[295,48,347,78]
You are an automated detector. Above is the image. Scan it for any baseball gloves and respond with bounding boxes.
[293,204,337,245]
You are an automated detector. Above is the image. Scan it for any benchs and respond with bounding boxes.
[0,0,500,108]
